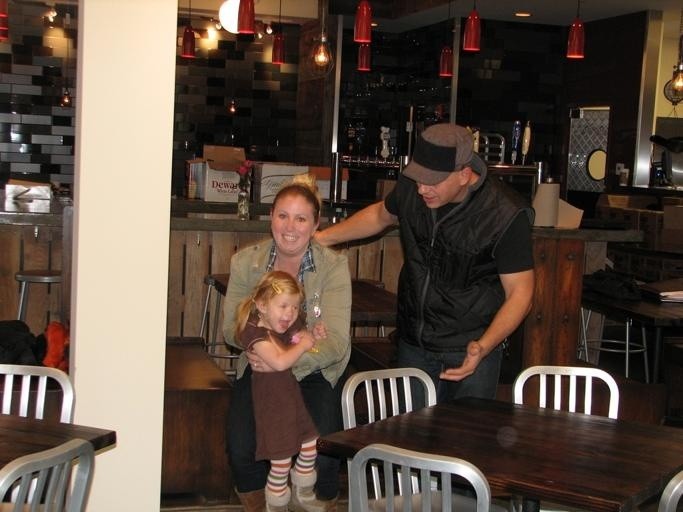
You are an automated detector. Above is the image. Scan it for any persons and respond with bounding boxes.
[235,270,329,512]
[312,122,536,415]
[223,184,352,512]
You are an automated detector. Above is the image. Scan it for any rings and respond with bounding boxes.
[254,361,257,367]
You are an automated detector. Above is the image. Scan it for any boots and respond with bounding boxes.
[290,469,326,511]
[234,485,265,512]
[265,486,291,512]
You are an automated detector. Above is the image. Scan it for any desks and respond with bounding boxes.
[345,280,398,339]
[582,278,683,383]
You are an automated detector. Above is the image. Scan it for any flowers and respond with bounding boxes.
[232,159,257,189]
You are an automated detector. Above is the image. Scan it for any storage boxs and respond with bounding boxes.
[307,165,350,202]
[252,160,310,205]
[199,143,251,204]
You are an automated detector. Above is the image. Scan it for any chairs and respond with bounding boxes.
[0,363,117,512]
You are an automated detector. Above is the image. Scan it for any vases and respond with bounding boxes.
[236,186,250,224]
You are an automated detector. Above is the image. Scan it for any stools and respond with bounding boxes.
[578,305,649,383]
[199,272,241,377]
[13,271,64,332]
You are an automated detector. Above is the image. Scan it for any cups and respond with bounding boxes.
[618,168,630,187]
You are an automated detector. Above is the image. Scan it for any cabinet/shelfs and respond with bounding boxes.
[159,335,238,501]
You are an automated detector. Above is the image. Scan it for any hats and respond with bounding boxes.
[403,124,474,186]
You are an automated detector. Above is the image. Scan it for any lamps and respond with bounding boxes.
[462,0,481,53]
[59,38,72,108]
[352,0,373,73]
[271,1,287,65]
[438,0,454,79]
[303,1,335,79]
[0,0,10,42]
[564,0,587,59]
[663,61,683,107]
[237,0,258,35]
[179,0,197,59]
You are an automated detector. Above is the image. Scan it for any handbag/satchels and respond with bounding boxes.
[582,264,641,302]
[0,319,46,366]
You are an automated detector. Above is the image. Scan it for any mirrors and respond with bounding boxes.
[586,149,606,181]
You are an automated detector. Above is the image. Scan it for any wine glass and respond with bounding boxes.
[389,145,399,165]
